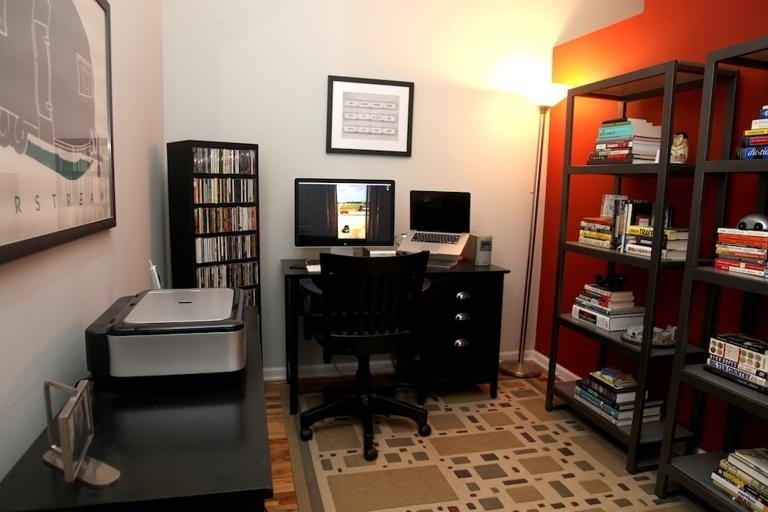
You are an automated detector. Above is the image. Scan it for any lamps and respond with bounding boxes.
[500,82,570,379]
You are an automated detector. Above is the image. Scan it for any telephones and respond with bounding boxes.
[149,259,164,290]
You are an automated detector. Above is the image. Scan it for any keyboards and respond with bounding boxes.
[306,264,321,272]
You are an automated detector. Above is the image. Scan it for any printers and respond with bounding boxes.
[84,284,248,389]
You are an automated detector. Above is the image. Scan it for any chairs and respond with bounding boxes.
[300,250,432,462]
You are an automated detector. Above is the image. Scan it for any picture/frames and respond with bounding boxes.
[56,379,98,483]
[1,1,118,263]
[325,75,415,158]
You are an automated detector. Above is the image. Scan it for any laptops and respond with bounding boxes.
[396,190,471,256]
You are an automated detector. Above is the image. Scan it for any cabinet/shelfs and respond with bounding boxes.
[165,139,263,368]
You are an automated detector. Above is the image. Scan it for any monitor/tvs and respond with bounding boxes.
[292,178,396,255]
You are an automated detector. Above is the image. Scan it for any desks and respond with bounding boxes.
[280,256,510,415]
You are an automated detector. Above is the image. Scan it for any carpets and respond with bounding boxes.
[280,367,702,511]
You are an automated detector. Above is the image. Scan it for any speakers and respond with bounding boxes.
[463,232,493,267]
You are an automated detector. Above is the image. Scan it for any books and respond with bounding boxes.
[735,103,768,161]
[574,369,662,428]
[578,192,689,262]
[709,448,767,512]
[713,226,768,282]
[193,146,258,307]
[586,117,661,165]
[703,330,768,396]
[570,273,647,332]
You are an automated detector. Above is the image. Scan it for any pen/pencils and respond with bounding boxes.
[289,266,304,269]
